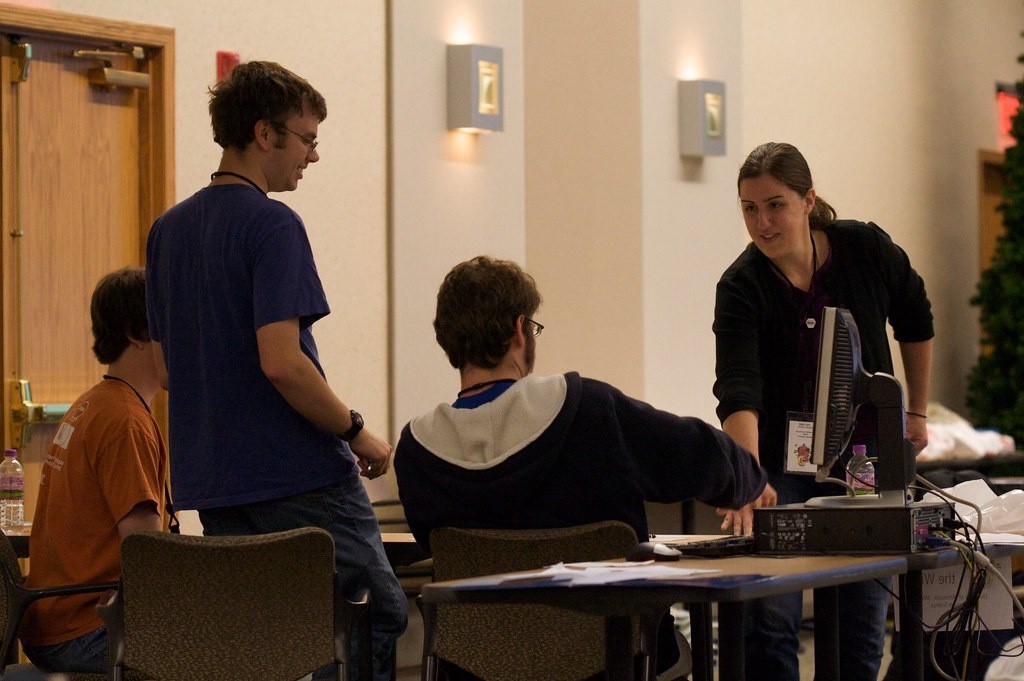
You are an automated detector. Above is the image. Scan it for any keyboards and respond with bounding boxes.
[661,533,752,557]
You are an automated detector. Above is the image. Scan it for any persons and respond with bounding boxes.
[19,267,171,670]
[394,254,768,681]
[711,142,935,681]
[147,60,409,681]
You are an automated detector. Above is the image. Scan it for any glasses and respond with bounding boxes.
[513,314,544,337]
[251,117,319,152]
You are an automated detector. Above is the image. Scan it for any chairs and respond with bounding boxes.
[0,528,376,681]
[425,523,691,680]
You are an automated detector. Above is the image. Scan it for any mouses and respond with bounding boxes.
[625,542,682,563]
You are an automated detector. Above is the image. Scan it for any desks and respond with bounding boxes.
[421,538,1024,681]
[3,526,422,565]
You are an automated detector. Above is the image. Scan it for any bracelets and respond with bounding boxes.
[906,412,927,419]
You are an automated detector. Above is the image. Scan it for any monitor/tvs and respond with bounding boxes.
[809,306,917,510]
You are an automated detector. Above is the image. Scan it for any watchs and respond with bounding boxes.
[340,409,365,441]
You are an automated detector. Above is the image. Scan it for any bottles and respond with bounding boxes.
[0,449,25,535]
[845,445,876,496]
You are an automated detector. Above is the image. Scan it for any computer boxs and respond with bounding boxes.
[749,501,956,558]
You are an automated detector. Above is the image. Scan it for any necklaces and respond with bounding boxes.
[211,171,267,196]
[457,377,515,397]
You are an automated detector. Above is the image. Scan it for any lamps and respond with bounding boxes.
[679,79,728,156]
[447,44,503,134]
